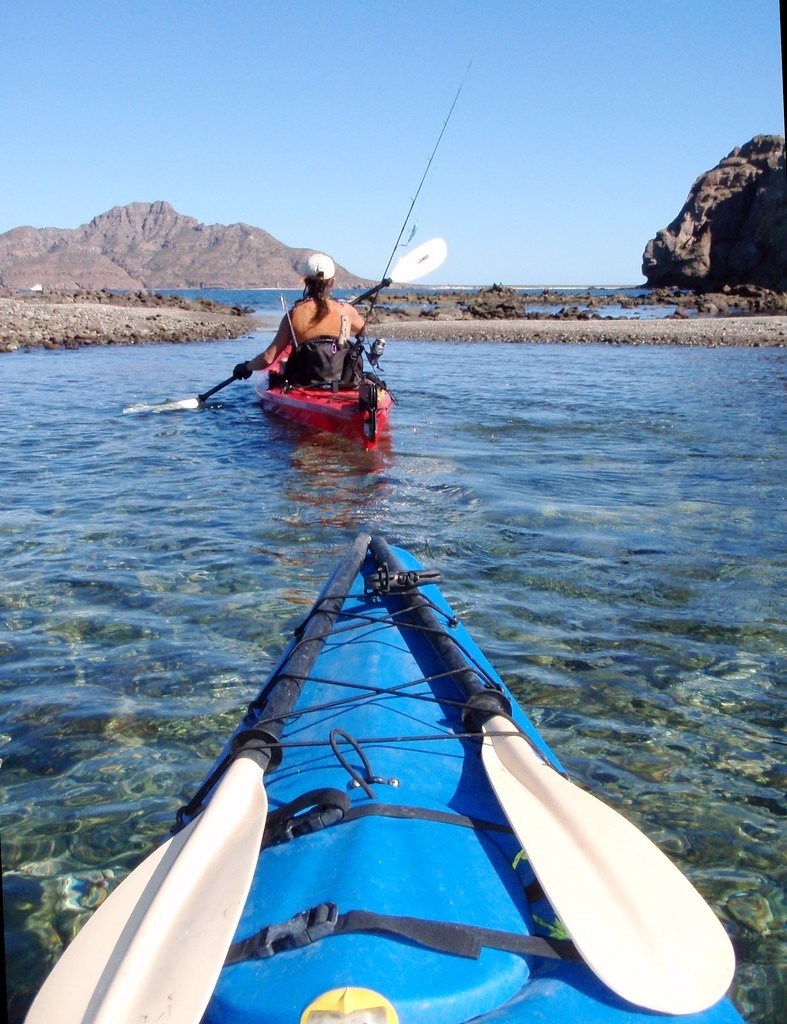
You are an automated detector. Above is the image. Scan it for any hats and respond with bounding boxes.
[303,254,335,280]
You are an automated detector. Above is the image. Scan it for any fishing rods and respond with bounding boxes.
[360,61,473,349]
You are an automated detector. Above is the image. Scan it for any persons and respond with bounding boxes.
[233,253,364,387]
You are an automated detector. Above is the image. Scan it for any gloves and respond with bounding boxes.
[233,360,253,380]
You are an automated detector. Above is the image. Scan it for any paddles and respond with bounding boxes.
[131,237,447,412]
[378,536,738,1017]
[16,536,374,1023]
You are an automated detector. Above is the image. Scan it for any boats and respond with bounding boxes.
[254,340,395,451]
[24,533,746,1024]
